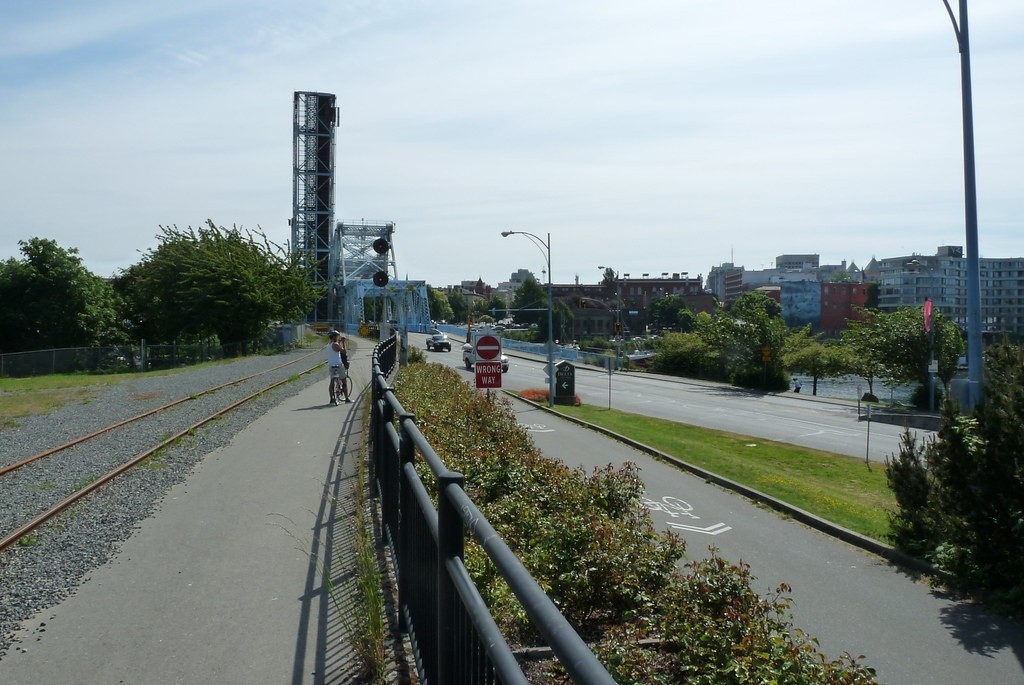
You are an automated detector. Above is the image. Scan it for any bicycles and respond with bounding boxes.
[329,359,353,406]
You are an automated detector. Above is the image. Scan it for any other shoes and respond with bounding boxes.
[345,399,356,404]
[330,399,338,405]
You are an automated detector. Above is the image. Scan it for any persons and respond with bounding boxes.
[435,322,437,329]
[793,378,801,393]
[327,330,355,404]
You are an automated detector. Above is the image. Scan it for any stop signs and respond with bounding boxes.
[475,335,502,361]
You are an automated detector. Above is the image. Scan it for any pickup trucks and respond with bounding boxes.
[463,346,509,372]
[425,334,452,352]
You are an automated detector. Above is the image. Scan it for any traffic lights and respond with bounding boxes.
[615,323,621,332]
[374,271,388,287]
[373,238,389,255]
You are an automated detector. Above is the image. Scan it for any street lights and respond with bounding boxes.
[582,297,620,370]
[500,230,555,407]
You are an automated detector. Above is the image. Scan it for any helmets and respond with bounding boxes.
[328,331,339,337]
